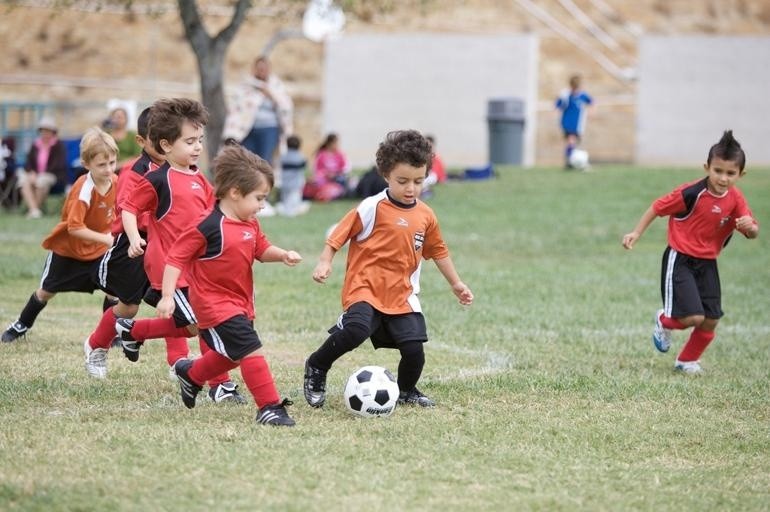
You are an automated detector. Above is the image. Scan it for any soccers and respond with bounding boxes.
[345,366,400,418]
[569,149,588,169]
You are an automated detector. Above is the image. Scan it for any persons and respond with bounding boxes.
[85,106,190,386]
[303,128,474,408]
[555,77,594,166]
[116,95,245,405]
[0,127,123,341]
[156,144,302,426]
[622,129,759,376]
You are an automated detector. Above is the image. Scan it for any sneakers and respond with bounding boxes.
[83,317,143,379]
[653,306,705,373]
[399,389,433,409]
[1,322,30,341]
[303,357,328,407]
[175,359,294,429]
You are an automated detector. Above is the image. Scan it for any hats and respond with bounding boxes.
[37,115,59,134]
